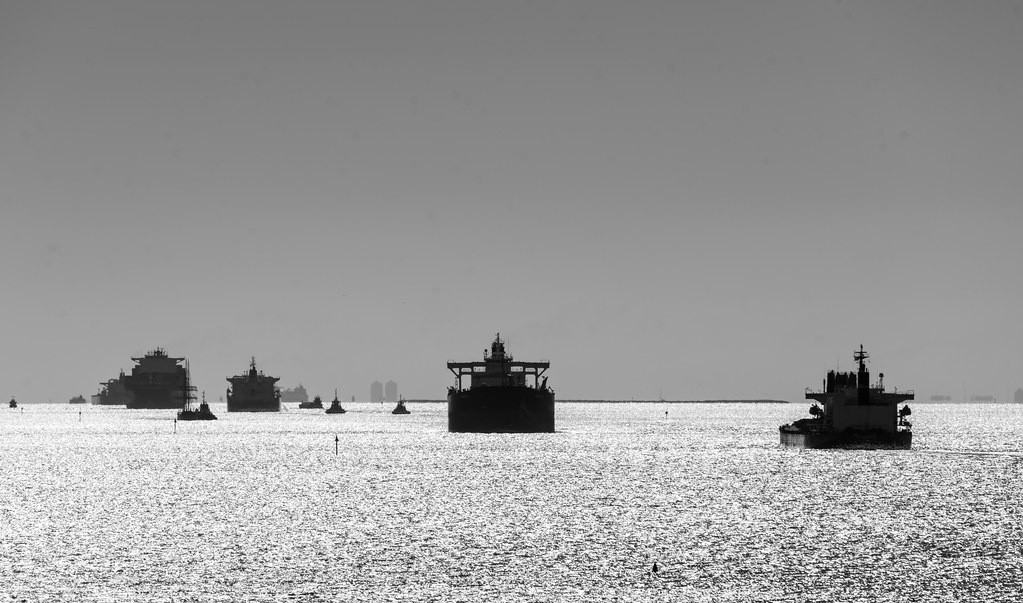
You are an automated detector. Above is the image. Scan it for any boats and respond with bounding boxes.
[70,394,86,404]
[780,344,913,448]
[177,391,217,420]
[92,346,199,408]
[225,356,281,412]
[9,396,18,407]
[392,395,411,414]
[299,395,323,408]
[325,387,347,413]
[447,333,556,431]
